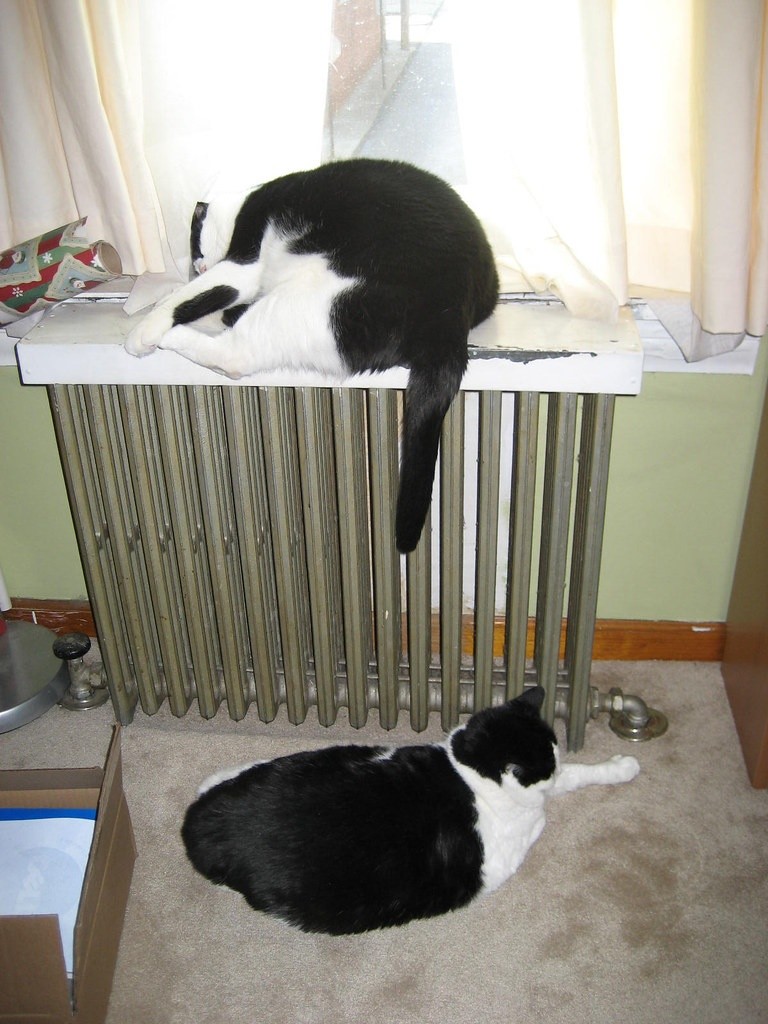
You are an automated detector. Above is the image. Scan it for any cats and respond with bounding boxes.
[124,158,500,554]
[180,685,641,938]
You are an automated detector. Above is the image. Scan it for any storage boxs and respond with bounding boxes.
[0,720,138,1024]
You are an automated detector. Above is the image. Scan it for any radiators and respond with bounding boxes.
[46,383,648,757]
